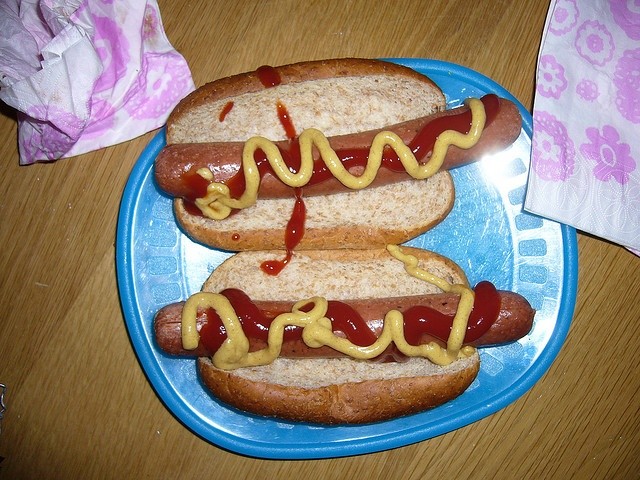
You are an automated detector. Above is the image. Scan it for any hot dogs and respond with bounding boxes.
[153,57,521,248]
[150,245,537,424]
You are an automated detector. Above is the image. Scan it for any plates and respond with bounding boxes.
[115,59,578,460]
